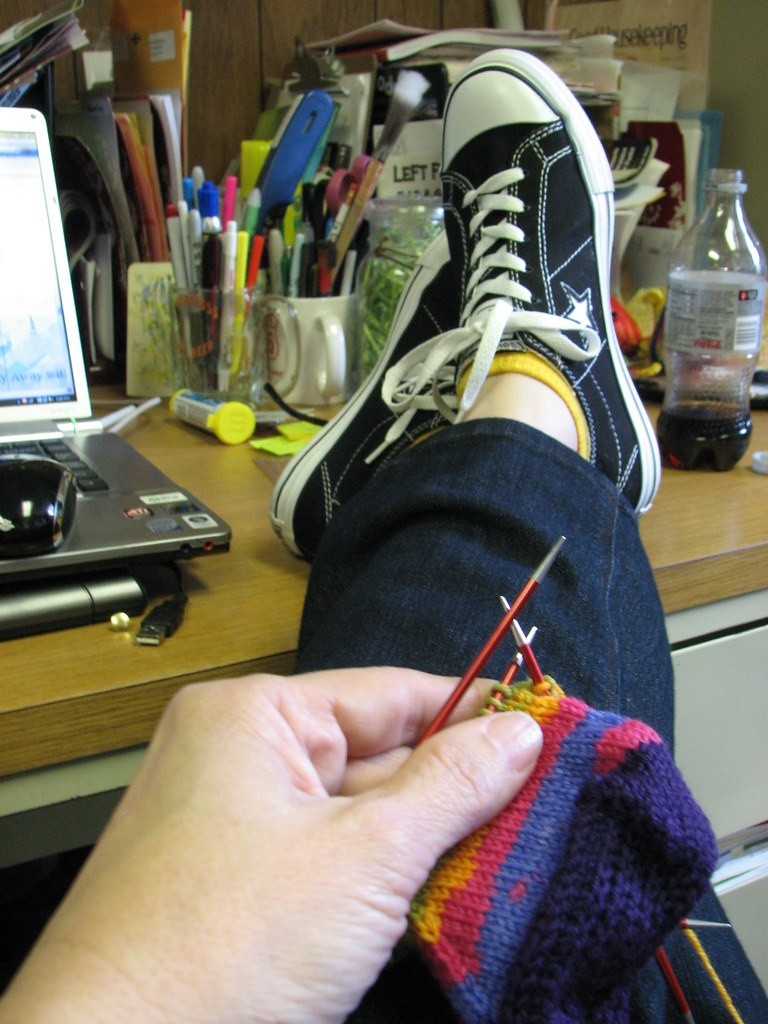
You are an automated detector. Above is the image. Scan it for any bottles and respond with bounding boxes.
[657,166,768,470]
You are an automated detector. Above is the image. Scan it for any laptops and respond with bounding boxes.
[0,104,234,640]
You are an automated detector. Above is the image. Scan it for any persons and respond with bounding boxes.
[0,48,768,1023]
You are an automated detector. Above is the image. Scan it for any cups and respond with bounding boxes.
[170,286,304,400]
[267,294,358,408]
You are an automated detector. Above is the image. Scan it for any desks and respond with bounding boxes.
[1,380,767,1024]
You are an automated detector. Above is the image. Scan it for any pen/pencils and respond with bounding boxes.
[162,68,431,411]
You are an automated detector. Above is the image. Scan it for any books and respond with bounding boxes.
[0,2,730,389]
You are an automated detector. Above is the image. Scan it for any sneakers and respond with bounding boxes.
[362,47,662,519]
[265,229,463,567]
